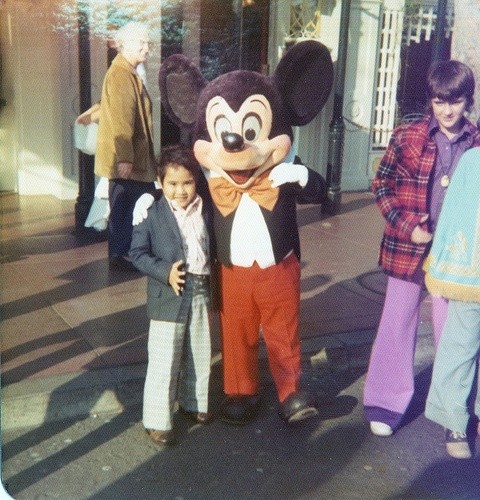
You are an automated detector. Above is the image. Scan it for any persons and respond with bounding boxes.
[363,60,480,436]
[93,22,156,270]
[423,147,480,459]
[129,143,217,442]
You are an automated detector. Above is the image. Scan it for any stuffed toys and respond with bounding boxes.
[158,40,333,423]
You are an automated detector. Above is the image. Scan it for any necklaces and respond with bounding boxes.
[433,132,460,187]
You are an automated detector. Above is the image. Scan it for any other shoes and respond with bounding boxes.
[444,427,472,459]
[144,424,176,445]
[110,249,134,263]
[181,404,213,425]
[370,419,393,436]
